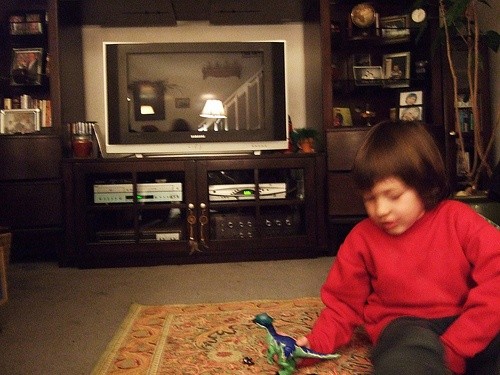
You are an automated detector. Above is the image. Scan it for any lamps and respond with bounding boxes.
[199,98,228,119]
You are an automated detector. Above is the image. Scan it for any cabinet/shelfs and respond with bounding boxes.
[317,0,493,257]
[64,150,328,269]
[0,0,84,267]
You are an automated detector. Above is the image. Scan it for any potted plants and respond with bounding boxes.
[290,127,325,153]
[412,0,500,224]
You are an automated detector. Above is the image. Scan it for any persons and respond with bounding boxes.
[331,15,423,126]
[271,121,500,375]
[7,117,36,134]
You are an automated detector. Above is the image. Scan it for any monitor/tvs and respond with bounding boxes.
[78,24,323,163]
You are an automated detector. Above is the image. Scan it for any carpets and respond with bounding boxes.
[88,297,375,375]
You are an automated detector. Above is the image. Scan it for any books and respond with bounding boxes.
[40,95,51,127]
[3,94,39,109]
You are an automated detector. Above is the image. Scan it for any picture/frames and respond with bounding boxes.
[395,88,424,122]
[381,50,411,88]
[378,13,413,45]
[10,47,42,87]
[0,109,41,135]
[351,64,383,87]
